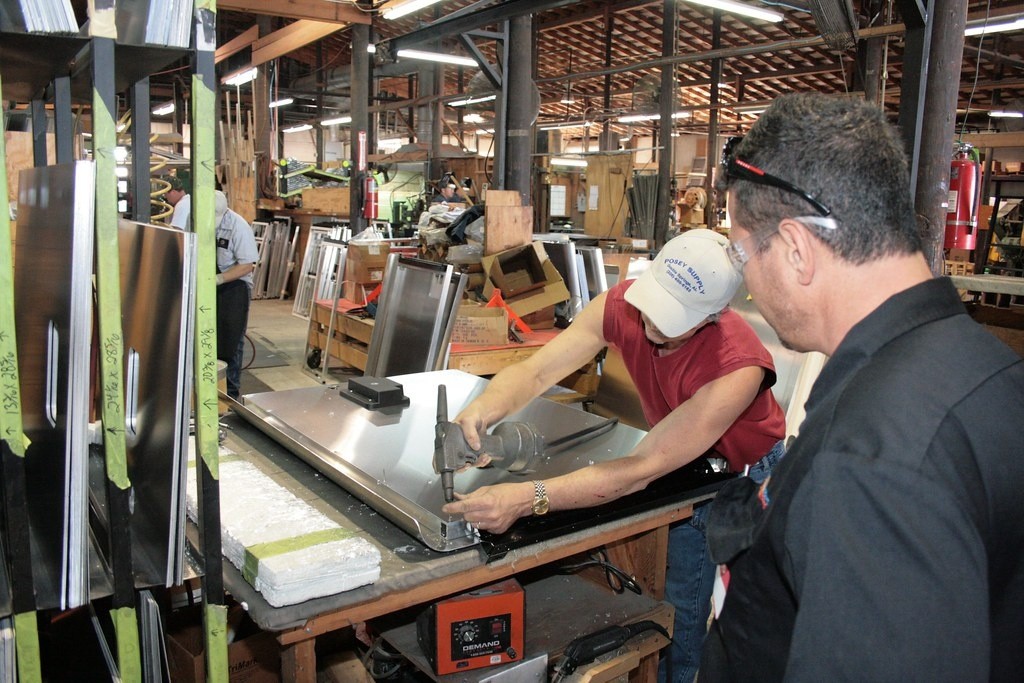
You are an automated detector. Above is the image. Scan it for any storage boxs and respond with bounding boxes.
[458,242,571,330]
[450,307,509,345]
[151,582,282,683]
[343,258,387,284]
[339,281,382,303]
[348,242,389,261]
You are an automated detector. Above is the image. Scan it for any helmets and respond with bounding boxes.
[438,172,456,191]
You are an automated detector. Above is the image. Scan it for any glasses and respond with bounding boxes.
[726,217,836,276]
[721,137,831,216]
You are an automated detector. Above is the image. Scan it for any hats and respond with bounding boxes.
[160,175,183,190]
[215,190,227,228]
[625,227,742,338]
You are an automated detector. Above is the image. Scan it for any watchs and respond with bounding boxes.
[533,479,549,517]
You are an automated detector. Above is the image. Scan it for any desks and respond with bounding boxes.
[186,413,693,683]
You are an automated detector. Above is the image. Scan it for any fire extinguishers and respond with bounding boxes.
[364,176,379,219]
[944,142,983,250]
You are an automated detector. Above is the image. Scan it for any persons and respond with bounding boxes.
[696,93,1024,683]
[166,177,260,401]
[444,229,789,683]
[432,180,471,207]
